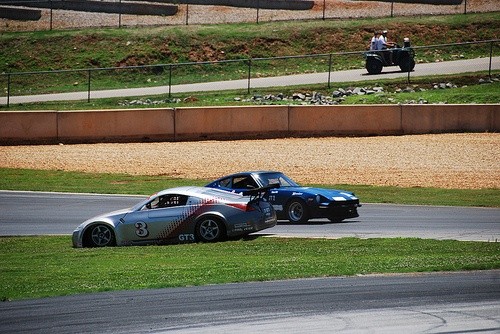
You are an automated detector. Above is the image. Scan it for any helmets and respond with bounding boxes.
[381,30,388,37]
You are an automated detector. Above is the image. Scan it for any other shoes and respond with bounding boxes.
[388,61,394,65]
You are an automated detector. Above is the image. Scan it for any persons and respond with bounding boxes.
[370,31,381,54]
[378,30,395,65]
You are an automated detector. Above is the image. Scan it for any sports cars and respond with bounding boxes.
[204,170,362,225]
[72,186,278,248]
